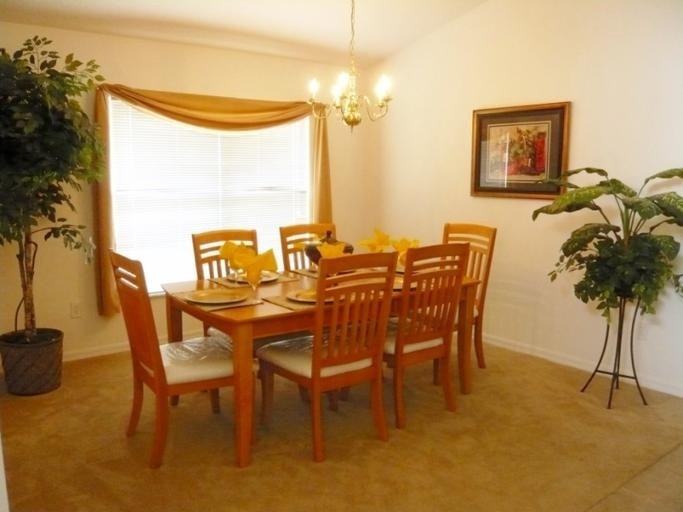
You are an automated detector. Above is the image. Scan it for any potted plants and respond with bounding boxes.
[530,166,683,321]
[0,35,111,397]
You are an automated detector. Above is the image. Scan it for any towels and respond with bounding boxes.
[220,228,424,284]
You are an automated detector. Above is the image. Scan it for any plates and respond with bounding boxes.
[227,270,280,282]
[184,288,249,304]
[287,288,345,302]
[393,276,417,290]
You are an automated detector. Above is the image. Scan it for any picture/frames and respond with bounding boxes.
[469,101,572,201]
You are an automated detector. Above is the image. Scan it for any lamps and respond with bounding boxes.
[302,0,396,132]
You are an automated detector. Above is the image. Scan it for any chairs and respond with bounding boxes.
[192,226,279,394]
[256,252,400,464]
[279,223,352,336]
[406,221,497,383]
[109,250,262,472]
[333,242,472,426]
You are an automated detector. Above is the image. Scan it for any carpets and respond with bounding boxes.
[0,327,683,512]
